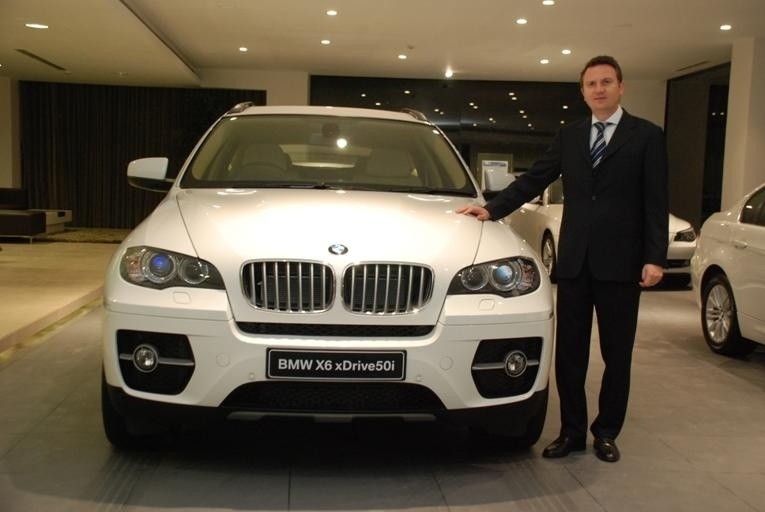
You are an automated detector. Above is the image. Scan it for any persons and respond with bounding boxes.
[455,54,671,463]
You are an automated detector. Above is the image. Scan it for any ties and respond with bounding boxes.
[588,121,612,170]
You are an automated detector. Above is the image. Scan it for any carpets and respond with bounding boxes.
[32,228,134,244]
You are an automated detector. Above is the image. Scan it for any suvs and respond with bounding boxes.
[101,101,555,447]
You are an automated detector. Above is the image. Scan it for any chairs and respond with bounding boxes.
[0,186,45,244]
[352,144,424,187]
[227,140,289,181]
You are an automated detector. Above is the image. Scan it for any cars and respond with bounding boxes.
[501,173,697,284]
[690,183,765,354]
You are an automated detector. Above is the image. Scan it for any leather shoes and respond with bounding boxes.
[541,432,588,460]
[592,436,622,462]
